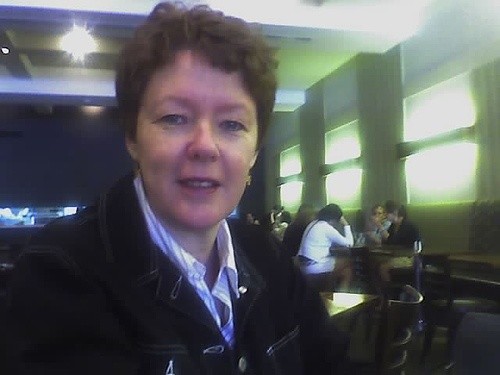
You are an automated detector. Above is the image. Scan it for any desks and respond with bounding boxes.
[317,291,381,349]
[330,243,415,257]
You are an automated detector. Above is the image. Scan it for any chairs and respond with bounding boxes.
[433,312,500,375]
[351,285,424,375]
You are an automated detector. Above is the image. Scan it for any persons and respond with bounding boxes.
[250,198,426,344]
[0,0,350,375]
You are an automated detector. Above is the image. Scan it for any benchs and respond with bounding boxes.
[285,199,500,285]
